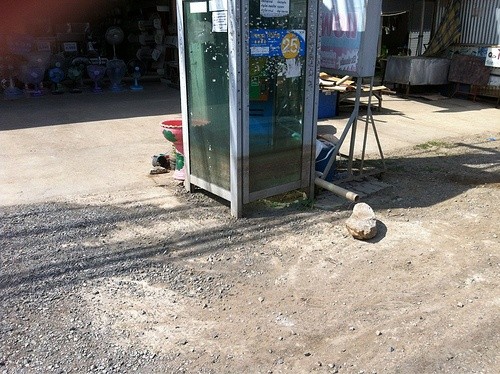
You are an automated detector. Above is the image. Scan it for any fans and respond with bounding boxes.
[68,56,91,85]
[125,61,146,93]
[48,66,66,92]
[65,67,88,94]
[106,62,127,94]
[86,65,105,94]
[17,53,51,97]
[105,26,124,58]
[0,57,21,97]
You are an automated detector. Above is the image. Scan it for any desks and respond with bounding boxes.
[446,54,491,104]
